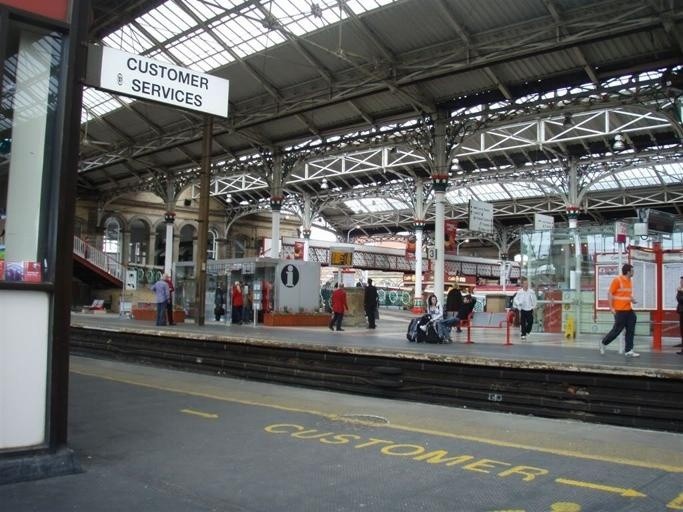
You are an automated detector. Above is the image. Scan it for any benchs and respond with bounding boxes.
[443,310,516,346]
[82,299,106,315]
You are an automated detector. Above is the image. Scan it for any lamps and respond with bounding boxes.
[449,157,461,171]
[611,131,625,151]
[225,193,232,204]
[319,175,328,189]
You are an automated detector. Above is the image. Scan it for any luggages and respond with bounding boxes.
[406,315,441,343]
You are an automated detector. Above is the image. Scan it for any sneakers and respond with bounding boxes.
[624,349,640,357]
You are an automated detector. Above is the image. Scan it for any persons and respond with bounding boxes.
[229,278,273,325]
[598,264,641,359]
[673,276,683,355]
[436,294,474,343]
[150,273,171,326]
[213,279,223,323]
[338,254,345,265]
[446,283,464,311]
[355,278,366,287]
[362,277,378,329]
[165,272,175,326]
[512,275,537,340]
[327,282,348,331]
[320,282,332,313]
[419,294,443,336]
